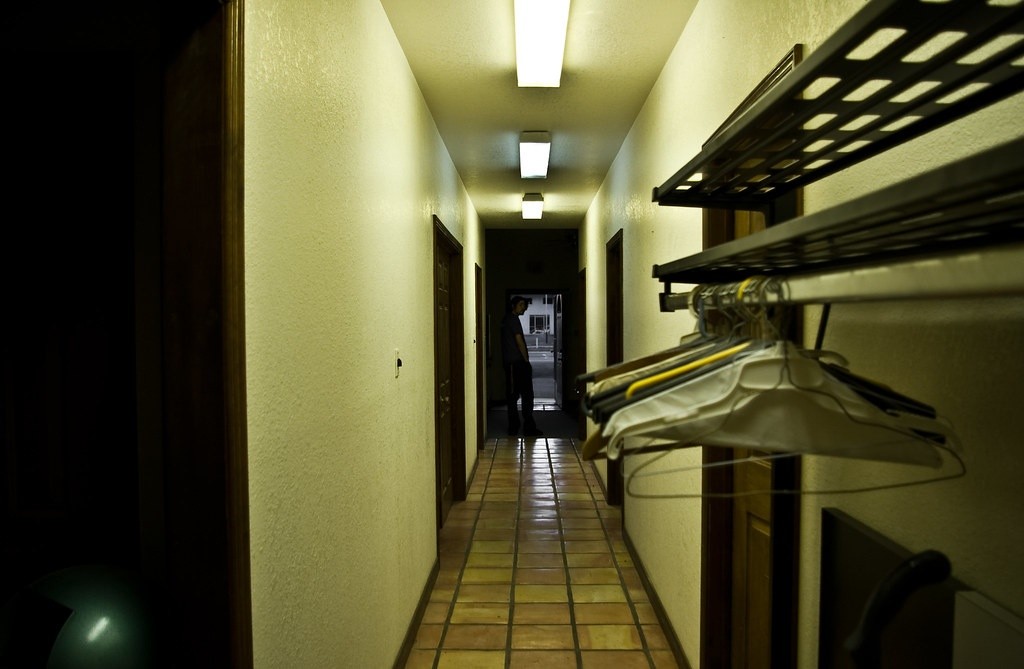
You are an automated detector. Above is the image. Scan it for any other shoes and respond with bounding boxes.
[508,426,518,435]
[523,429,543,437]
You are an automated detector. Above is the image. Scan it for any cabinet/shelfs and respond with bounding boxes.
[652,0,1024,320]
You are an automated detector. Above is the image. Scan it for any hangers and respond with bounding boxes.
[575,279,966,500]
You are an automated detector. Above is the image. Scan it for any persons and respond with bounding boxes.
[501,296,543,438]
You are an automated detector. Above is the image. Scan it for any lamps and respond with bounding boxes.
[521,192,544,220]
[513,0,571,87]
[519,129,552,179]
